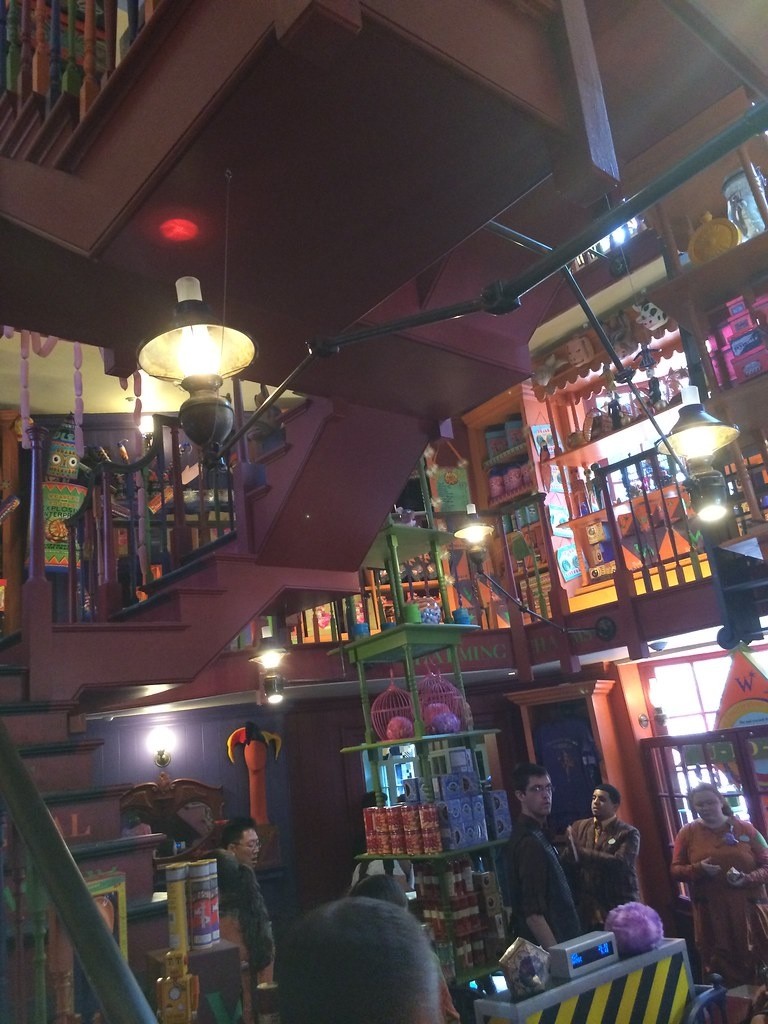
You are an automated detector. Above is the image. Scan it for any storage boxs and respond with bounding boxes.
[724,294,768,383]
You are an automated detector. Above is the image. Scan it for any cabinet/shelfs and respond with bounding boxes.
[619,81,767,562]
[530,253,768,614]
[326,455,511,1024]
[459,382,565,623]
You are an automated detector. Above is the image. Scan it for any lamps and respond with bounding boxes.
[134,276,255,473]
[638,681,672,728]
[655,403,739,522]
[452,504,494,572]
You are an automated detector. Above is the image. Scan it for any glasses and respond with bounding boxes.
[521,786,555,793]
[231,841,264,848]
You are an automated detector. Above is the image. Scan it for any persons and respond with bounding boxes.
[670,784,768,990]
[496,763,641,949]
[195,814,462,1023]
